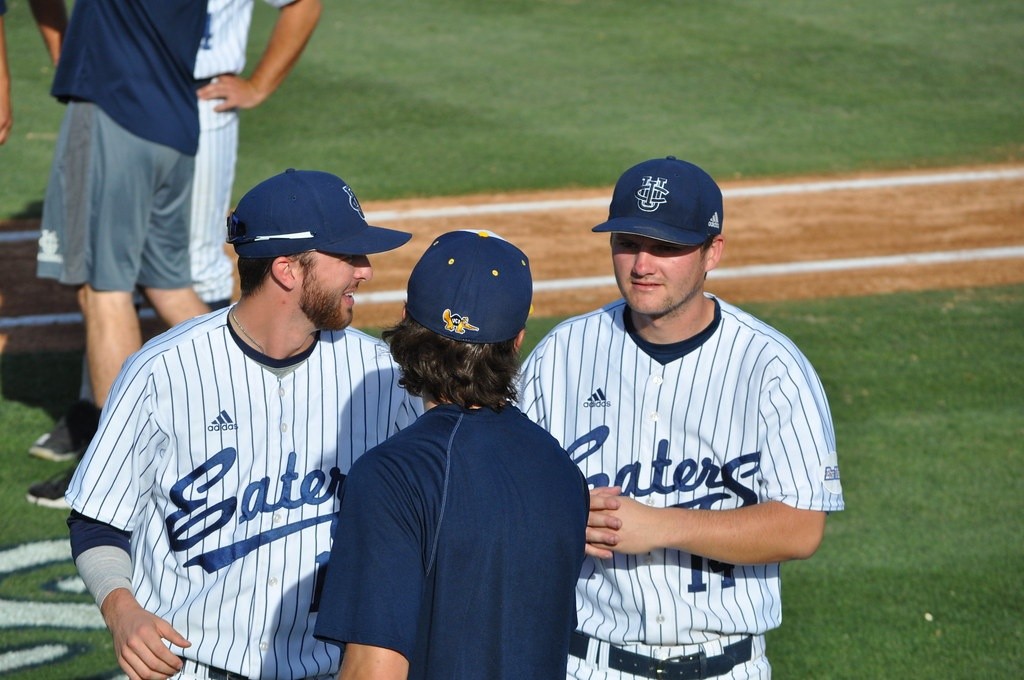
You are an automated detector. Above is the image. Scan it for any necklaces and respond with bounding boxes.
[233,308,263,352]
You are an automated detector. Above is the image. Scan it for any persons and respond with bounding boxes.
[66,169,423,680]
[513,157,844,680]
[314,230,591,680]
[0,0,13,144]
[27,0,321,460]
[24,0,209,511]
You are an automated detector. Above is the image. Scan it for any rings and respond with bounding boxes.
[211,76,219,84]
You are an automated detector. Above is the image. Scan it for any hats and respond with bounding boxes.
[591,156,723,246]
[234,168,411,258]
[407,229,534,341]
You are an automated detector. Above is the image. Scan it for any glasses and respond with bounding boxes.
[226,212,315,245]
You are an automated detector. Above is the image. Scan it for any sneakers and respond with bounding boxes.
[28,402,95,460]
[26,466,80,509]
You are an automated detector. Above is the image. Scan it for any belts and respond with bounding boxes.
[176,654,248,680]
[569,626,751,679]
